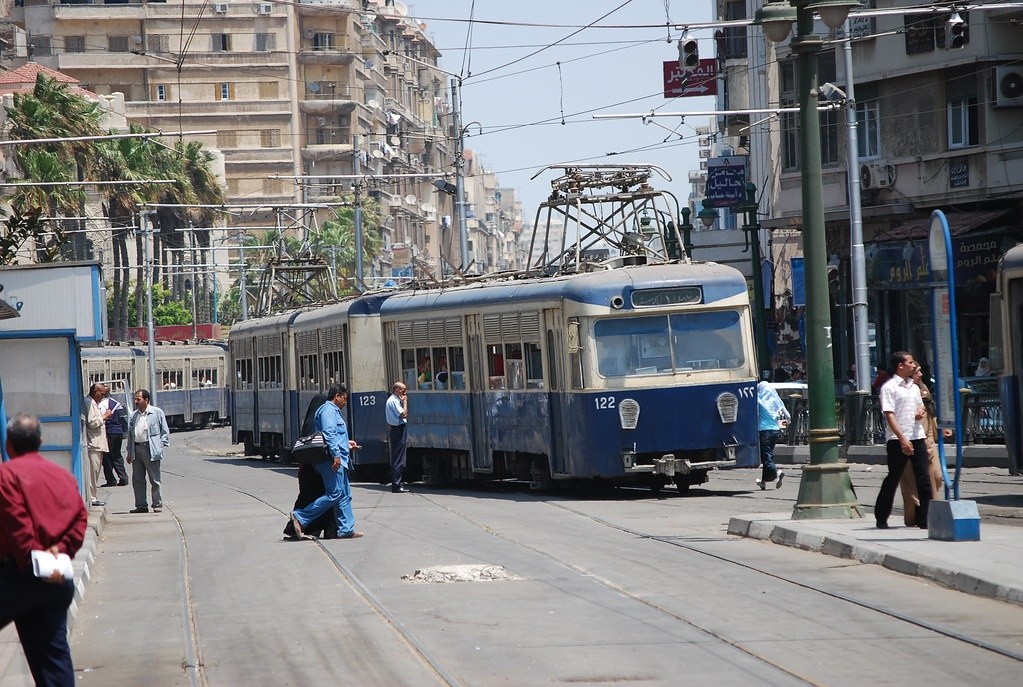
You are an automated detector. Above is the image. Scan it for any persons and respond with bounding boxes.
[874,351,932,529]
[385,381,412,494]
[896,361,942,528]
[290,384,363,540]
[780,358,993,444]
[0,414,87,687]
[285,394,340,539]
[85,382,113,506]
[100,384,128,488]
[418,358,458,389]
[126,389,169,513]
[164,376,214,390]
[755,375,792,489]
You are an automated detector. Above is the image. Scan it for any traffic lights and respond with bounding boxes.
[679,39,700,72]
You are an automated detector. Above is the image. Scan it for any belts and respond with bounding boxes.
[136,443,148,445]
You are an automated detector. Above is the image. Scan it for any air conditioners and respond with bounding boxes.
[859,159,896,189]
[258,4,273,15]
[216,3,229,13]
[991,64,1023,109]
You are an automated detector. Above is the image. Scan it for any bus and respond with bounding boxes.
[226,260,761,496]
[990,244,1023,475]
[80,342,230,431]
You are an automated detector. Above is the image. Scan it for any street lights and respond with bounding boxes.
[747,0,867,521]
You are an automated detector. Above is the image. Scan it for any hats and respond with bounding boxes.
[910,360,921,377]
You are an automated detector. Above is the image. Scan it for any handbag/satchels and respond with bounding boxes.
[291,405,330,464]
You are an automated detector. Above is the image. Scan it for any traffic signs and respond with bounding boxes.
[663,58,718,97]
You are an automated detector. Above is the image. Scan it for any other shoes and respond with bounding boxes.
[101,484,116,487]
[920,525,928,529]
[776,469,785,489]
[117,483,128,486]
[92,501,106,506]
[392,487,410,493]
[290,512,303,541]
[756,479,766,490]
[339,531,364,538]
[130,509,148,513]
[154,508,162,512]
[877,519,889,528]
